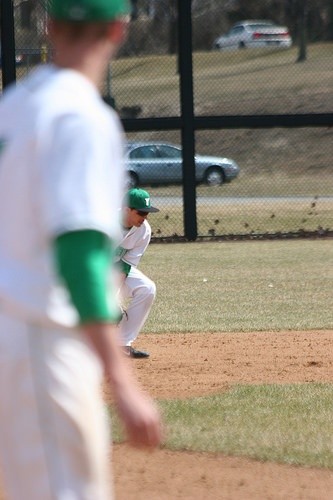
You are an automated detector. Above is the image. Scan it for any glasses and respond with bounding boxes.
[137,212,149,216]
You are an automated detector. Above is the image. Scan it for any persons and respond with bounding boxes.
[0,1,163,500]
[108,188,161,358]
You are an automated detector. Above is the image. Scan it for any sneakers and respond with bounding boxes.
[120,346,149,358]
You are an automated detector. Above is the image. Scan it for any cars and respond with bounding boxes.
[211,21,292,50]
[124,142,241,190]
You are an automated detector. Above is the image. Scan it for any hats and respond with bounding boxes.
[123,188,160,212]
[49,1,133,22]
